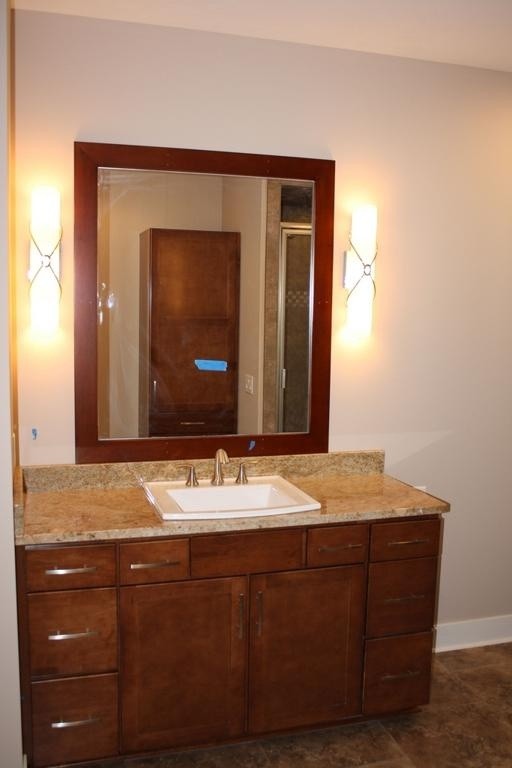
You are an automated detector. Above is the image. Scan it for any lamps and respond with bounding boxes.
[342,202,379,344]
[28,186,61,340]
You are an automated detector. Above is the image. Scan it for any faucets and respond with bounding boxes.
[211,448,230,486]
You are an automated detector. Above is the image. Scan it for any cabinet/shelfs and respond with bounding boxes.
[138,228,242,439]
[19,543,120,768]
[121,520,369,760]
[361,517,444,720]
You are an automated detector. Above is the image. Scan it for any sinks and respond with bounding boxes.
[166,482,292,514]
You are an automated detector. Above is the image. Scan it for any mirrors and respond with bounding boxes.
[73,140,335,466]
